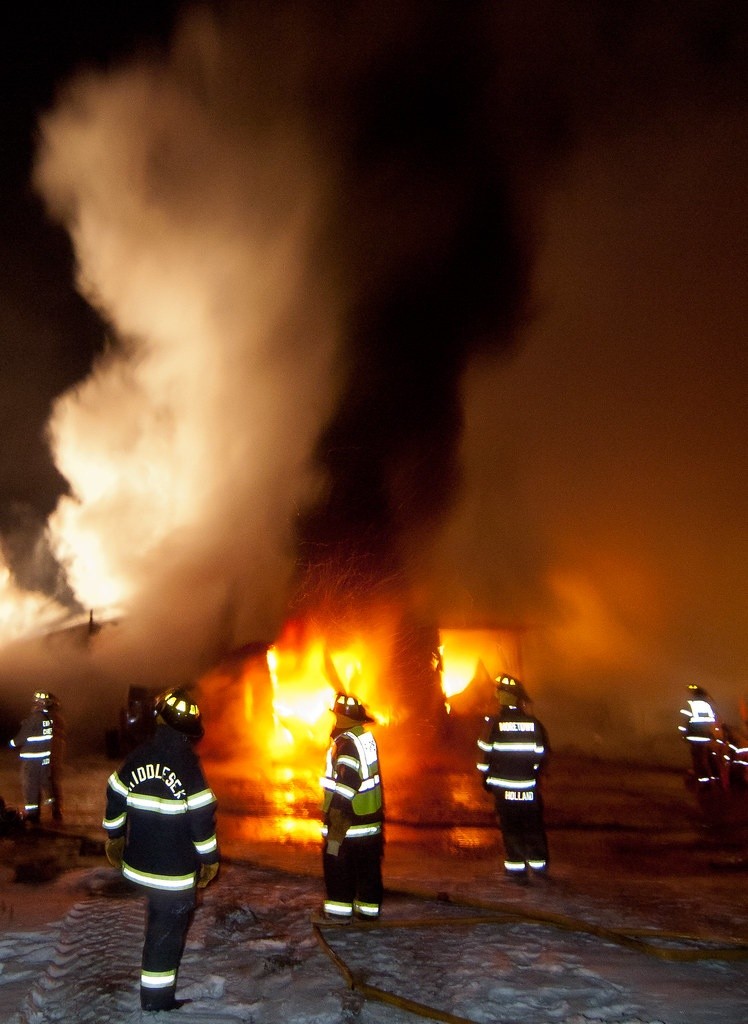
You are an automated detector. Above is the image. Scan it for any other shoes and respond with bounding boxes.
[532,864,547,874]
[353,911,378,921]
[51,808,61,819]
[503,869,528,877]
[310,908,350,924]
[23,812,41,824]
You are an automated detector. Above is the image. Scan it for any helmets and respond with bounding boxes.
[687,684,706,696]
[32,690,50,707]
[494,673,525,698]
[329,694,373,721]
[155,687,202,737]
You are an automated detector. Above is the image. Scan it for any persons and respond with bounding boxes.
[475,675,548,879]
[103,691,220,1011]
[9,691,63,827]
[318,694,385,923]
[679,684,725,799]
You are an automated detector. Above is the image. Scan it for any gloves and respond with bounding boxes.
[482,773,492,793]
[105,837,125,869]
[195,863,220,888]
[9,743,14,749]
